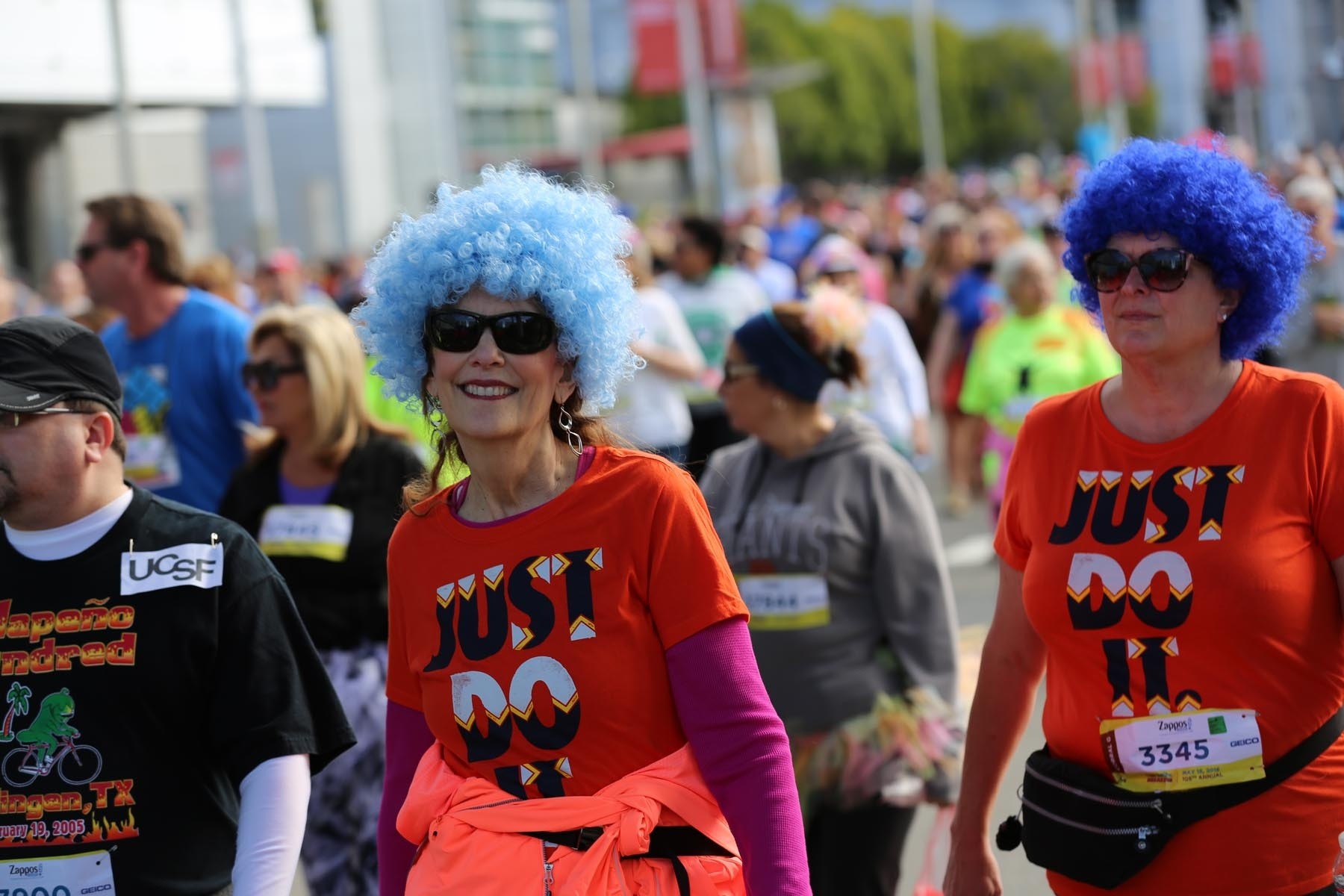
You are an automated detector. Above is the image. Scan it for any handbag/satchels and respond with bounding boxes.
[995,708,1341,890]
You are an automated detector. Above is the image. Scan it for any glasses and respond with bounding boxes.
[241,357,304,392]
[1085,248,1194,293]
[1,404,100,424]
[427,309,559,355]
[720,361,761,382]
[77,233,123,261]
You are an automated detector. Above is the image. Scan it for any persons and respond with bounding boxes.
[942,137,1344,896]
[213,299,441,896]
[697,305,961,896]
[0,313,358,896]
[346,162,812,896]
[0,132,1344,527]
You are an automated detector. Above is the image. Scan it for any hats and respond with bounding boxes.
[1,312,123,418]
